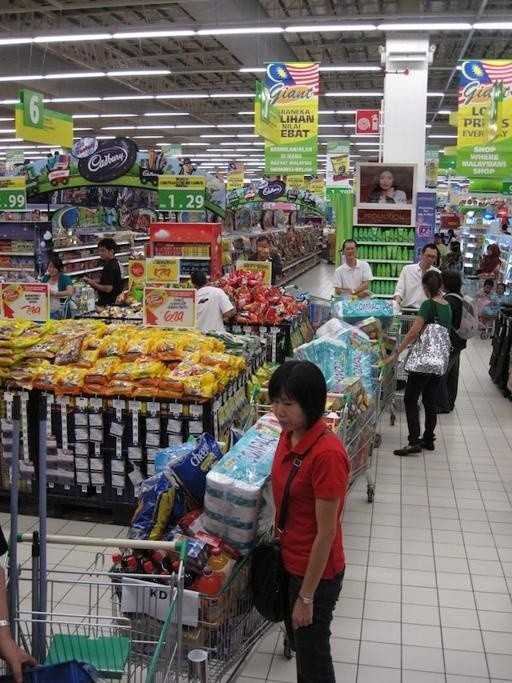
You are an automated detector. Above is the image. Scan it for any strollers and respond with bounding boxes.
[469,271,506,340]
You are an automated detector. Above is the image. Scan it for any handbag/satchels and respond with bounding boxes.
[69,296,83,310]
[251,540,294,622]
[403,320,452,378]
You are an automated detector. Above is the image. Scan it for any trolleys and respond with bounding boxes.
[251,307,424,504]
[110,525,297,683]
[0,532,184,682]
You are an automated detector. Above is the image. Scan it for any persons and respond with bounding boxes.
[367,164,405,204]
[392,243,441,392]
[334,238,376,297]
[437,270,468,413]
[30,208,43,223]
[434,198,512,334]
[250,237,282,286]
[136,214,150,227]
[43,255,73,318]
[379,272,452,457]
[0,520,40,682]
[180,157,192,176]
[83,237,123,305]
[268,360,353,682]
[191,269,237,336]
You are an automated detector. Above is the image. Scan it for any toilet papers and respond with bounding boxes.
[294,317,373,402]
[334,299,401,319]
[201,412,289,549]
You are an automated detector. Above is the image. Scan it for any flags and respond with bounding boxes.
[263,63,319,100]
[457,59,512,105]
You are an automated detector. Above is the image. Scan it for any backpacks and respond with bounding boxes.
[444,293,479,339]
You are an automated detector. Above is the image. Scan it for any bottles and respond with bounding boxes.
[107,548,231,623]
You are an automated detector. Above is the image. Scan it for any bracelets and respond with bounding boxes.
[0,619,10,626]
[297,593,314,605]
[55,291,59,296]
[395,349,401,359]
[352,288,356,294]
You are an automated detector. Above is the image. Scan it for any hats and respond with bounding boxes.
[435,233,442,238]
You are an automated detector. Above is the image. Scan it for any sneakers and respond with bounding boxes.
[420,439,434,450]
[397,445,424,456]
